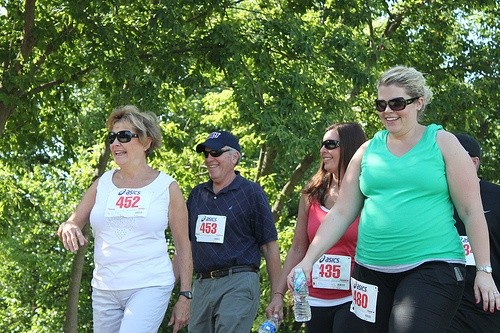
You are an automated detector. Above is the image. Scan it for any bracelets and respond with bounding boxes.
[273,291,285,298]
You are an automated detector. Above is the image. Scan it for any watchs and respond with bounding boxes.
[179,291,192,299]
[476,265,492,273]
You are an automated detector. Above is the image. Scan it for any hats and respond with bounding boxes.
[455,134,481,159]
[196,131,241,153]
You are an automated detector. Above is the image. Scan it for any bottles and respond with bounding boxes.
[292,268,311,322]
[258,314,279,333]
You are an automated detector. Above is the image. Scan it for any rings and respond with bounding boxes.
[494,291,500,295]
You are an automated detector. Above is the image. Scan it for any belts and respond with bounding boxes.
[196,265,258,280]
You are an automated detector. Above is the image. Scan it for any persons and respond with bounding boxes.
[58,104,194,333]
[266,67,500,333]
[172,131,284,333]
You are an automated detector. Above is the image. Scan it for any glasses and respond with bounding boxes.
[375,97,419,112]
[108,130,139,144]
[321,139,341,149]
[204,149,232,158]
